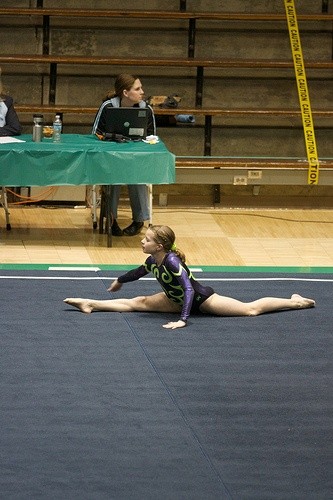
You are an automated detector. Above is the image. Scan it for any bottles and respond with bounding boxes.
[52,115,62,143]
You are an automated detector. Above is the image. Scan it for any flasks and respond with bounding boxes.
[31,114,43,142]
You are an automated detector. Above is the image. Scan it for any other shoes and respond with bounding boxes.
[123,221,144,235]
[111,219,123,236]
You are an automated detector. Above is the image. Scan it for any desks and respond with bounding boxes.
[0,134,177,248]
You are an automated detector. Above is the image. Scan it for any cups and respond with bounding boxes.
[42,126,52,139]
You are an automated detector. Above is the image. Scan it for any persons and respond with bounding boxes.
[62,225,316,330]
[91,74,156,237]
[0,66,22,137]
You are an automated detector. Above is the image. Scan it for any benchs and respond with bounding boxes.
[0,0,333,205]
